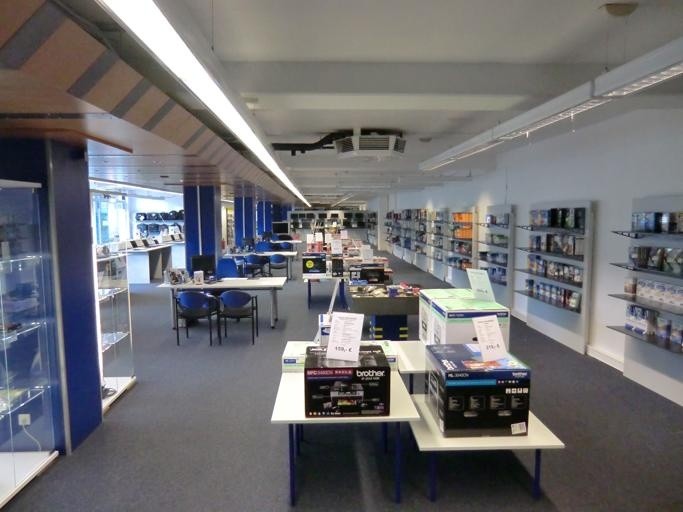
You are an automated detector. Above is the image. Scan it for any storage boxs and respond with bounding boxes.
[431,298,511,353]
[302,253,326,273]
[348,265,361,280]
[332,258,343,277]
[424,344,532,438]
[360,263,384,284]
[419,288,474,345]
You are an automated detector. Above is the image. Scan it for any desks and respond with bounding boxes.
[303,272,349,310]
[408,393,565,503]
[345,283,423,341]
[270,340,434,507]
[127,245,172,284]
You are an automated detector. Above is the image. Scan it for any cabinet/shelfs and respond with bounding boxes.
[0,179,60,509]
[287,210,378,243]
[512,200,595,356]
[384,206,515,308]
[607,193,683,406]
[89,188,137,416]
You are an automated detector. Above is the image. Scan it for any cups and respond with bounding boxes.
[194,271,203,285]
[245,273,254,279]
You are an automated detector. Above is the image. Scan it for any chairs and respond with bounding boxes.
[157,234,301,348]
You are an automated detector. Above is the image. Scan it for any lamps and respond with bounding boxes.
[414,36,682,171]
